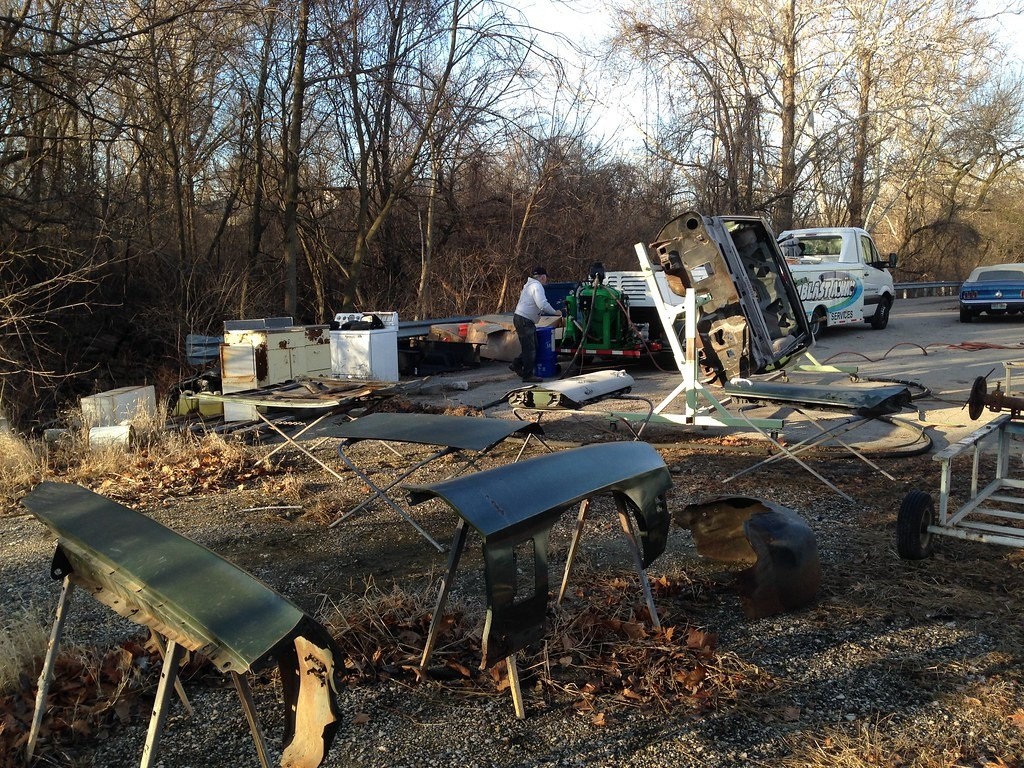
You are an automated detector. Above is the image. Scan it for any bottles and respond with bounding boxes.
[446,381,469,390]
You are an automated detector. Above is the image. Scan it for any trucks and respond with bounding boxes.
[602,227,898,343]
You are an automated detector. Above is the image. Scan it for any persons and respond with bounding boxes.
[508,267,561,382]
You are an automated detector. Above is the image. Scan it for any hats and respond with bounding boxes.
[532,267,549,278]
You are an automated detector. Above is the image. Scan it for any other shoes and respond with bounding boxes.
[509,364,524,376]
[522,376,543,382]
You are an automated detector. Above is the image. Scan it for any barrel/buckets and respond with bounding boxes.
[88,425,136,453]
[177,390,222,416]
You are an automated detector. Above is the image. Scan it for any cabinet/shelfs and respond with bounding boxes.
[220,324,331,421]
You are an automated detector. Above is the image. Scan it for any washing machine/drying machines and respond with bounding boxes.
[329,311,399,382]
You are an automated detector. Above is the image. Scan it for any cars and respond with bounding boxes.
[959,263,1024,322]
